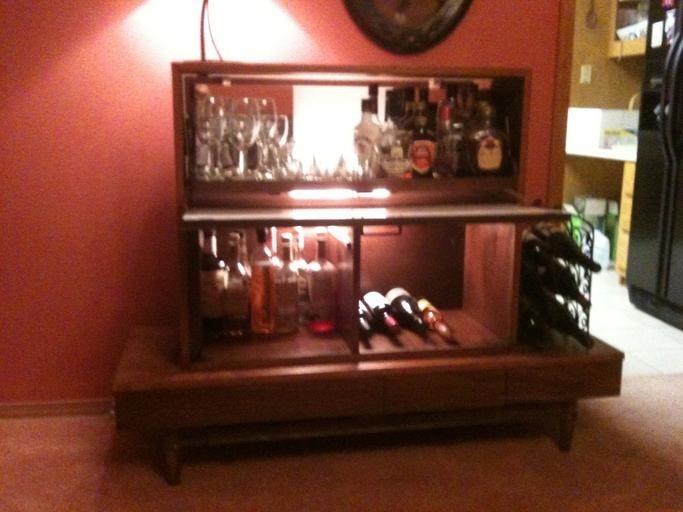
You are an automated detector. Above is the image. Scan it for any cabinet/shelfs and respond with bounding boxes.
[171,60,574,372]
[616,163,636,284]
[604,0,648,60]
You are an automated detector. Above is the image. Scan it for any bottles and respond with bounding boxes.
[353,80,511,180]
[197,226,339,337]
[519,220,602,350]
[358,288,453,345]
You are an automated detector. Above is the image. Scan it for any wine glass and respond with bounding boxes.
[190,96,289,182]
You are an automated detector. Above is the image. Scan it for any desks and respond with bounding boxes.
[113,327,624,485]
